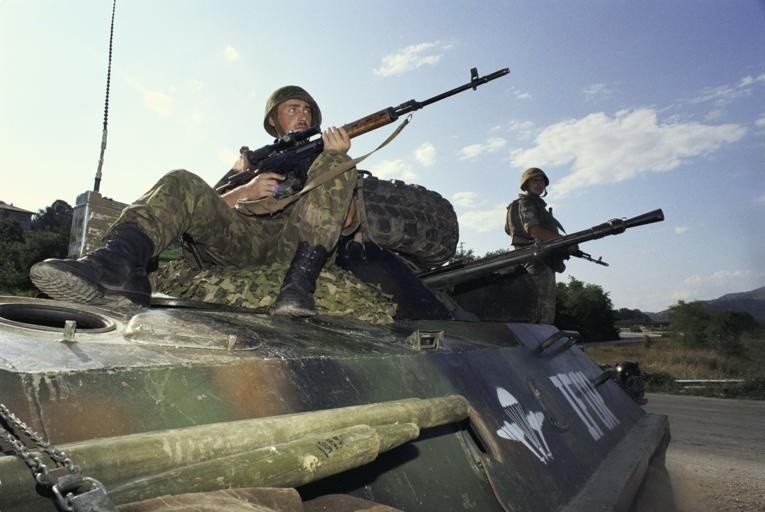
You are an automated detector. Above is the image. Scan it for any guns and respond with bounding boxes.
[417,208,664,288]
[214,67,510,200]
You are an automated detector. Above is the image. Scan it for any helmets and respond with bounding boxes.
[520,168,549,192]
[263,85,323,136]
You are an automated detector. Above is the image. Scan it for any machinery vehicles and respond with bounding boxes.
[1,0,675,509]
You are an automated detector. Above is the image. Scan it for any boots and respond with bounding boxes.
[30,224,156,309]
[271,241,330,319]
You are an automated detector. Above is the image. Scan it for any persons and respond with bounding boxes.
[510,167,581,327]
[27,84,361,321]
